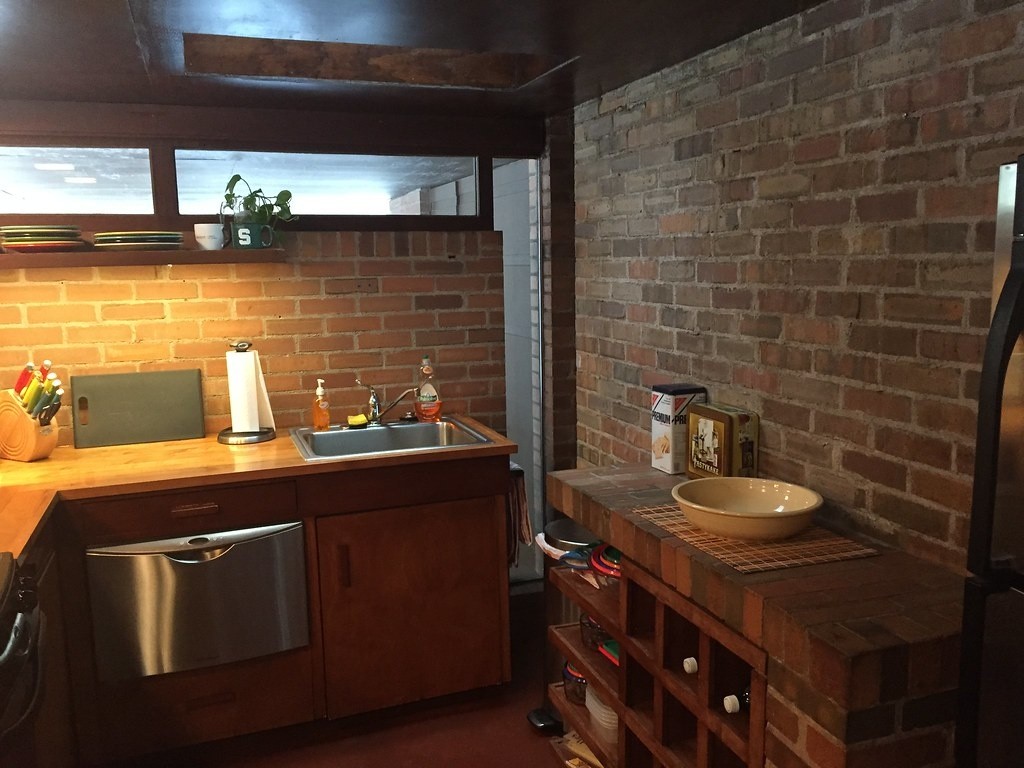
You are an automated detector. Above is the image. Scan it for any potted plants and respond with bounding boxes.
[220,174,300,248]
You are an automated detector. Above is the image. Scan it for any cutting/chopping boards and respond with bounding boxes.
[70,368,204,448]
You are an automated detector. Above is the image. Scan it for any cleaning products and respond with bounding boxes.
[311,377,330,431]
[414,354,442,422]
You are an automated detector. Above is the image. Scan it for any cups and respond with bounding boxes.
[231,223,273,249]
[194,223,232,250]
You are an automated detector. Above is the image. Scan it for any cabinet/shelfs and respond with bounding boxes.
[544,558,767,768]
[314,494,511,721]
[0,508,101,768]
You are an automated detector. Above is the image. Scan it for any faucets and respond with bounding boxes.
[355,378,418,427]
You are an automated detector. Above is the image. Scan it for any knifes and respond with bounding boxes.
[14,360,64,427]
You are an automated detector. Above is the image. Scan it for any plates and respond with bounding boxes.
[93,232,184,250]
[0,225,87,253]
[585,683,618,731]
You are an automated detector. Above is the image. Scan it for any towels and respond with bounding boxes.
[507,461,533,569]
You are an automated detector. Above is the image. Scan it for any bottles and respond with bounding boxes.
[684,656,699,674]
[724,682,751,713]
[413,353,442,423]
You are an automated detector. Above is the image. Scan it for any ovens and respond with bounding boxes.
[83,521,311,684]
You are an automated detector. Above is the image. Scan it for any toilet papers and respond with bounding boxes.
[225,348,278,433]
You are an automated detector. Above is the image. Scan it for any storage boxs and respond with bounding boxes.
[685,400,760,479]
[651,382,705,475]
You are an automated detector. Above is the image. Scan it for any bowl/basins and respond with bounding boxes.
[579,612,610,652]
[671,476,824,541]
[561,662,587,706]
[589,541,620,603]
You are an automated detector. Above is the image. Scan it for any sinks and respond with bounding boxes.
[289,416,496,463]
